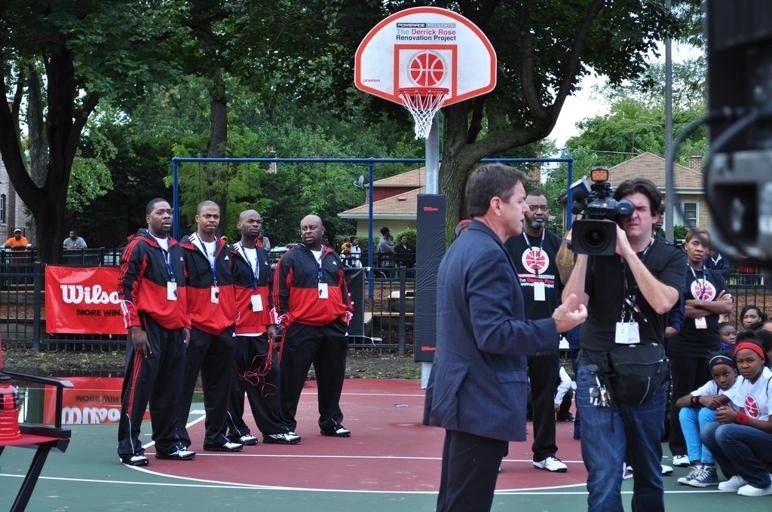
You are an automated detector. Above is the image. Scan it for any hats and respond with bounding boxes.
[268,246,289,268]
[96,250,121,266]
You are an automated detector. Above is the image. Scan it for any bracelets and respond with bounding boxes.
[736,411,750,425]
[691,396,697,408]
[695,395,702,407]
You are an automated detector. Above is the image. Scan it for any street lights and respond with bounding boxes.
[607,342,668,405]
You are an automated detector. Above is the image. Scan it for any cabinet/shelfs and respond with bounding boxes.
[0,375,23,443]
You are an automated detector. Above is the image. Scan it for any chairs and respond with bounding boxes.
[661,454,772,497]
[533,455,568,473]
[122,428,350,466]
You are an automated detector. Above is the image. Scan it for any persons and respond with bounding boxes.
[274,216,352,439]
[394,235,416,266]
[495,189,571,473]
[341,238,352,265]
[422,166,587,510]
[178,201,239,453]
[377,226,395,276]
[560,177,688,512]
[339,236,361,268]
[676,306,772,512]
[225,210,299,444]
[116,198,193,465]
[2,229,27,247]
[665,227,736,467]
[63,230,87,248]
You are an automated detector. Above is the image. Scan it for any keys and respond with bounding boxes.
[588,386,611,408]
